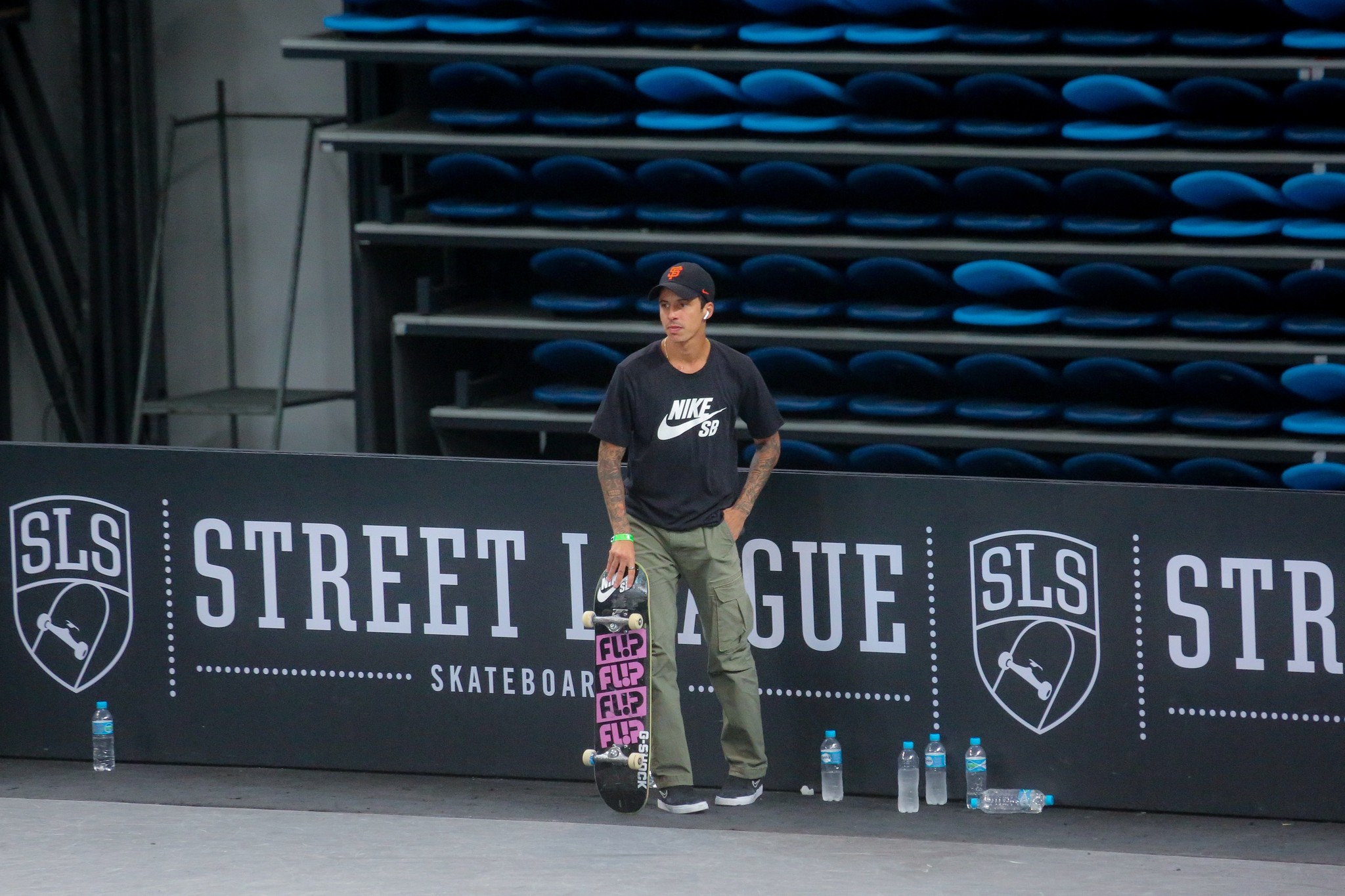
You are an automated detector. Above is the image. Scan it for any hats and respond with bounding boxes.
[648,263,715,302]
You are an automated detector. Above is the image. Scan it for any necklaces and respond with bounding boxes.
[664,336,669,358]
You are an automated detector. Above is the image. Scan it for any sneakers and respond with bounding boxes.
[657,785,709,814]
[715,775,763,806]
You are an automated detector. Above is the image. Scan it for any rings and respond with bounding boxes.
[628,567,636,569]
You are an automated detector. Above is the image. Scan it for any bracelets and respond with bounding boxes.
[610,534,634,544]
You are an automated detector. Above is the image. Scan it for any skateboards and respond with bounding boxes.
[582,562,654,814]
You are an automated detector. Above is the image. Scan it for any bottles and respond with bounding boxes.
[897,741,919,813]
[820,729,843,801]
[965,737,987,809]
[924,733,948,806]
[970,791,1043,814]
[982,788,1054,811]
[92,701,115,772]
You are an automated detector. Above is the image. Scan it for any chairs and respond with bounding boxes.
[278,0,1345,495]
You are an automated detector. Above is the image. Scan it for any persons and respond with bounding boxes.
[589,262,785,814]
